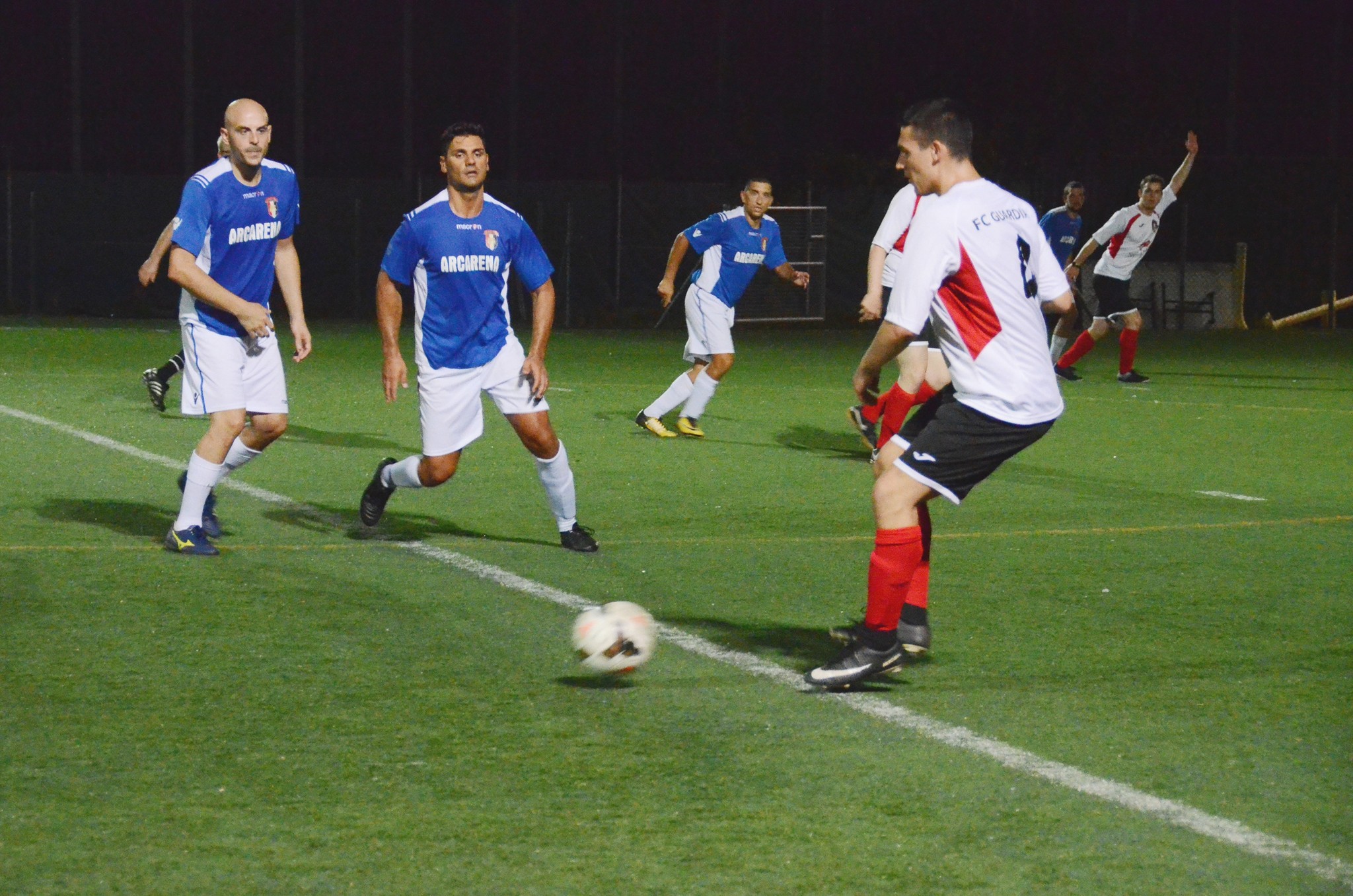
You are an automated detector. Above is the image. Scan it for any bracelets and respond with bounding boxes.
[1072,262,1081,269]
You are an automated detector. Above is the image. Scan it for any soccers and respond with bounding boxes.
[570,600,658,677]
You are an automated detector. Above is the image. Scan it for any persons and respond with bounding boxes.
[359,126,599,553]
[635,176,810,438]
[1052,129,1198,384]
[846,183,951,465]
[164,97,313,558]
[137,131,233,412]
[802,101,1075,686]
[1038,180,1086,376]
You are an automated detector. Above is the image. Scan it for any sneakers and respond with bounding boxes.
[560,520,599,552]
[166,524,220,557]
[674,415,707,438]
[1116,370,1150,384]
[830,618,932,658]
[142,369,169,412]
[176,471,221,538]
[635,409,677,438]
[361,457,400,527]
[846,404,879,449]
[1053,368,1084,382]
[803,640,904,689]
